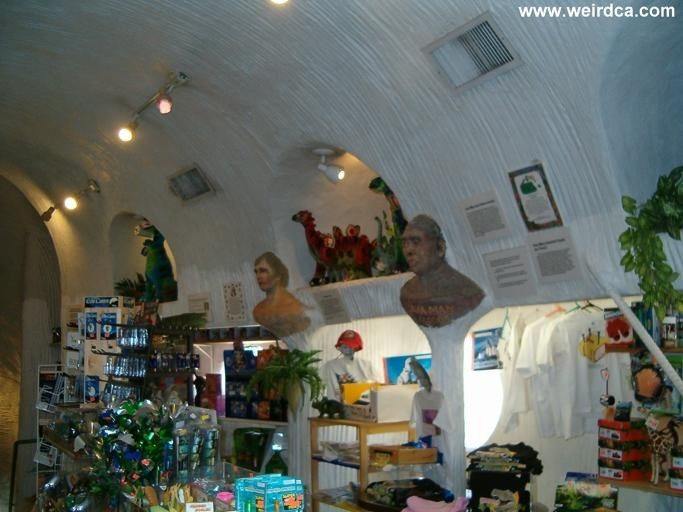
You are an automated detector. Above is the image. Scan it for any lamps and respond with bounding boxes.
[312,147,345,185]
[116,72,188,143]
[39,178,101,221]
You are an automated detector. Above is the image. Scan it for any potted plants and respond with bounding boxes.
[244,349,323,422]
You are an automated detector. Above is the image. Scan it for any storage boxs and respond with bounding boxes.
[337,381,419,424]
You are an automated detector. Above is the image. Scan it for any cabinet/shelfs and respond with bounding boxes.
[307,416,416,512]
[36,307,289,512]
[596,340,683,497]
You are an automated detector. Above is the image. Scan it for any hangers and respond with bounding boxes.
[499,297,604,345]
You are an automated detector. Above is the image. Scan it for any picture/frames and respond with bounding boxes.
[9,440,46,512]
[223,281,246,322]
[509,163,563,226]
[166,162,216,208]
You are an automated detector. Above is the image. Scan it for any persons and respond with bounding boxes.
[395,213,484,325]
[291,177,410,285]
[315,344,379,405]
[407,357,453,440]
[251,251,310,334]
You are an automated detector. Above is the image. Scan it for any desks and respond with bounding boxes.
[467,469,531,512]
